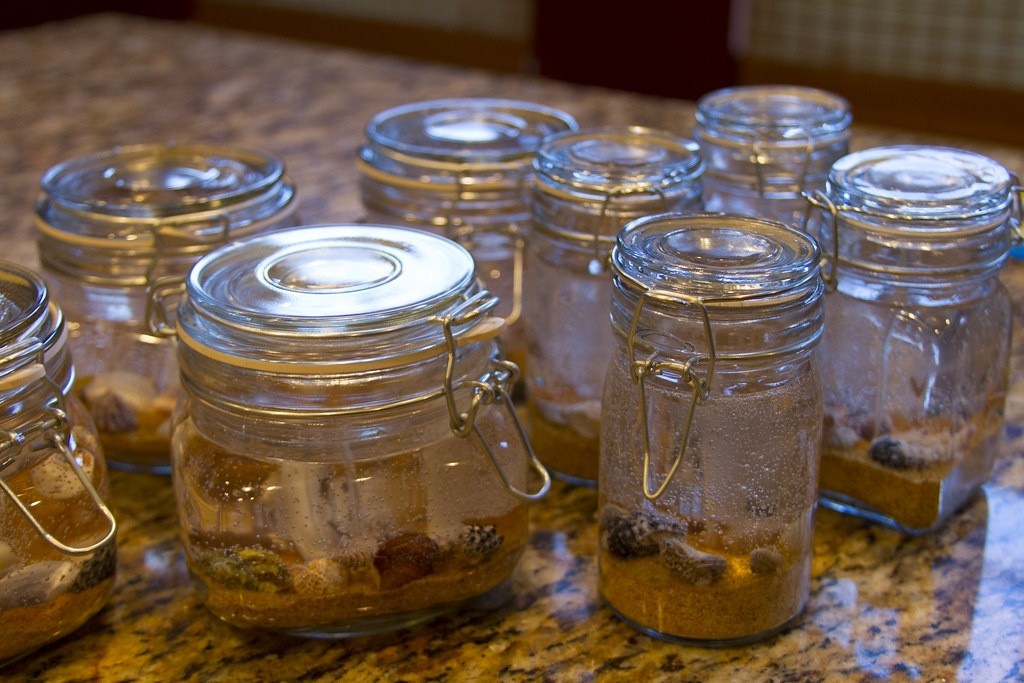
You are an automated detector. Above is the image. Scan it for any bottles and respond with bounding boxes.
[1,81,1021,670]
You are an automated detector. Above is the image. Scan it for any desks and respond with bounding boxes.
[0,15,1024,683]
